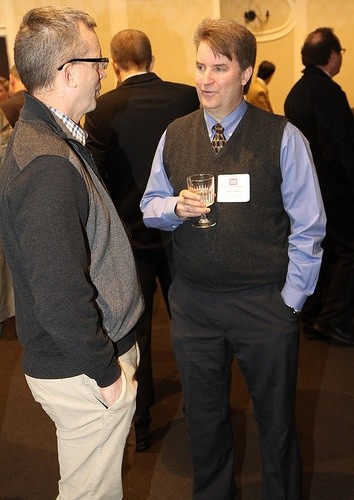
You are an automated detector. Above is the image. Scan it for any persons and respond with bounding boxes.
[0,5,145,500]
[138,17,328,500]
[83,29,200,452]
[0,63,29,173]
[247,26,353,350]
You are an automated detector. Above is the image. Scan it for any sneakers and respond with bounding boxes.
[136,427,151,451]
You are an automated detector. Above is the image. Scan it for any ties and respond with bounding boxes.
[211,123,227,154]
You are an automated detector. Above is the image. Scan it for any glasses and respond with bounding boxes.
[340,49,346,54]
[57,57,109,71]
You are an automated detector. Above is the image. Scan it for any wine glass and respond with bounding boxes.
[186,174,217,229]
